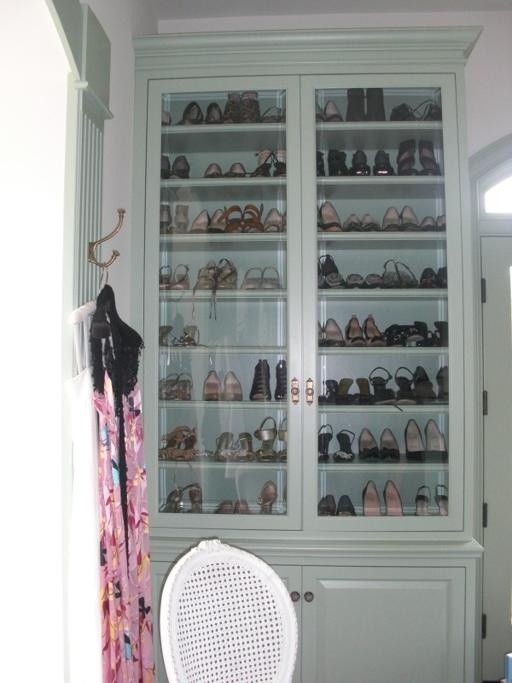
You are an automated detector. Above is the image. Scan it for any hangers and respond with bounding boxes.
[92,283,145,347]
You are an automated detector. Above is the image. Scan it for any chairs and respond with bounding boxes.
[156,539,299,682]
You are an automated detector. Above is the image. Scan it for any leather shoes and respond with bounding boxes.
[321,314,448,347]
[317,253,447,289]
[316,139,441,177]
[317,201,446,233]
[318,419,448,464]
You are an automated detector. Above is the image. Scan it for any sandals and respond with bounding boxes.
[159,359,287,400]
[322,365,449,405]
[161,203,287,234]
[161,146,287,179]
[162,92,285,126]
[159,258,282,290]
[159,480,278,514]
[316,87,442,123]
[159,324,199,347]
[317,480,448,517]
[157,415,288,462]
